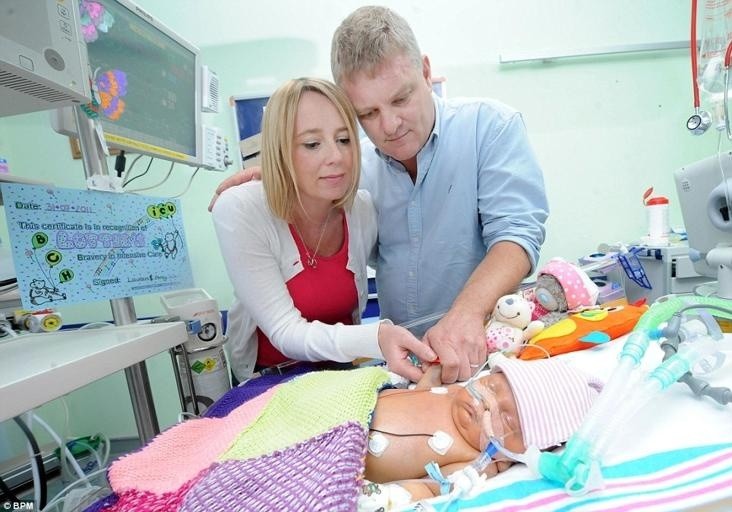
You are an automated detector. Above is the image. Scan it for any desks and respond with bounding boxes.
[0,321,197,512]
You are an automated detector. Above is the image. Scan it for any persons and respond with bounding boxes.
[211,76,439,385]
[207,6,550,385]
[363,366,527,507]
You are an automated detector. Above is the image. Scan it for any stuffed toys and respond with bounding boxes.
[481,291,543,359]
[521,259,600,331]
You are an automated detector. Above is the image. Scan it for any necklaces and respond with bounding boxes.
[292,210,330,269]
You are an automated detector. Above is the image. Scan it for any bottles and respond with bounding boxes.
[642,187,670,241]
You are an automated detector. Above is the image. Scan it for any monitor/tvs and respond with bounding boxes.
[50,0,204,168]
